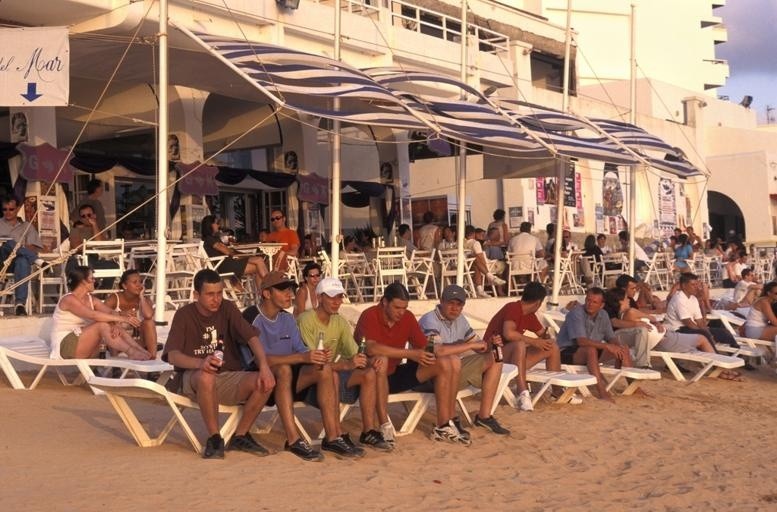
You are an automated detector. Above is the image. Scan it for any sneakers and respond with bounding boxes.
[474,414,510,436]
[147,372,160,380]
[16,307,27,315]
[518,390,534,412]
[430,417,471,447]
[203,436,225,459]
[548,392,583,404]
[487,276,505,285]
[322,433,365,458]
[359,428,397,453]
[476,291,491,298]
[284,438,324,461]
[228,433,268,457]
[41,262,48,274]
[111,369,122,378]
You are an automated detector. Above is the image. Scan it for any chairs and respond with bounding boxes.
[0,221,775,454]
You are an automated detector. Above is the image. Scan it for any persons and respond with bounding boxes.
[400,209,777,298]
[49,267,160,381]
[354,283,510,448]
[238,271,366,462]
[162,268,276,459]
[285,151,298,171]
[484,267,777,412]
[167,134,179,161]
[0,194,50,315]
[11,112,27,138]
[49,179,371,296]
[299,276,392,456]
[380,162,394,181]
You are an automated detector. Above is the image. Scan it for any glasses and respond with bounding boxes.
[3,208,15,211]
[80,213,91,218]
[309,273,320,277]
[271,216,282,222]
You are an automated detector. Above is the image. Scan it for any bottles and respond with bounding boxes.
[371,235,398,249]
[99,337,107,359]
[425,335,434,354]
[211,335,225,371]
[614,353,621,369]
[543,326,550,352]
[356,336,366,370]
[228,235,234,245]
[491,332,502,362]
[314,331,323,371]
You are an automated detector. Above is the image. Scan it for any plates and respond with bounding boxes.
[0,237,14,242]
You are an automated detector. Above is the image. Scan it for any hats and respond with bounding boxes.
[261,271,299,290]
[316,278,348,296]
[441,285,467,303]
